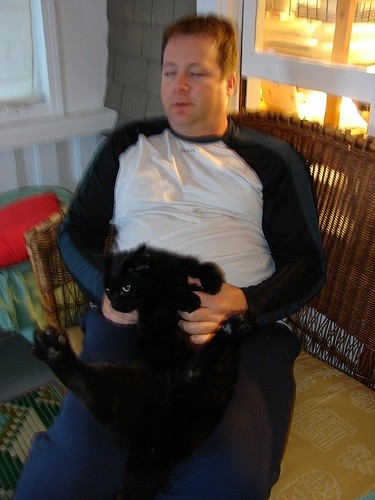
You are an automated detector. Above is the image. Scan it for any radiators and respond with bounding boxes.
[1,107,118,193]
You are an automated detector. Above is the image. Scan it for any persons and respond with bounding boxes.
[12,15,325,500]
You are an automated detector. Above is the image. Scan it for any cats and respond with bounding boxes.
[30,245,237,497]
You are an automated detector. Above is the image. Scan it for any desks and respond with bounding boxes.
[0,185,75,332]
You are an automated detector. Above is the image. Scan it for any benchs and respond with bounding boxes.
[22,110,375,500]
